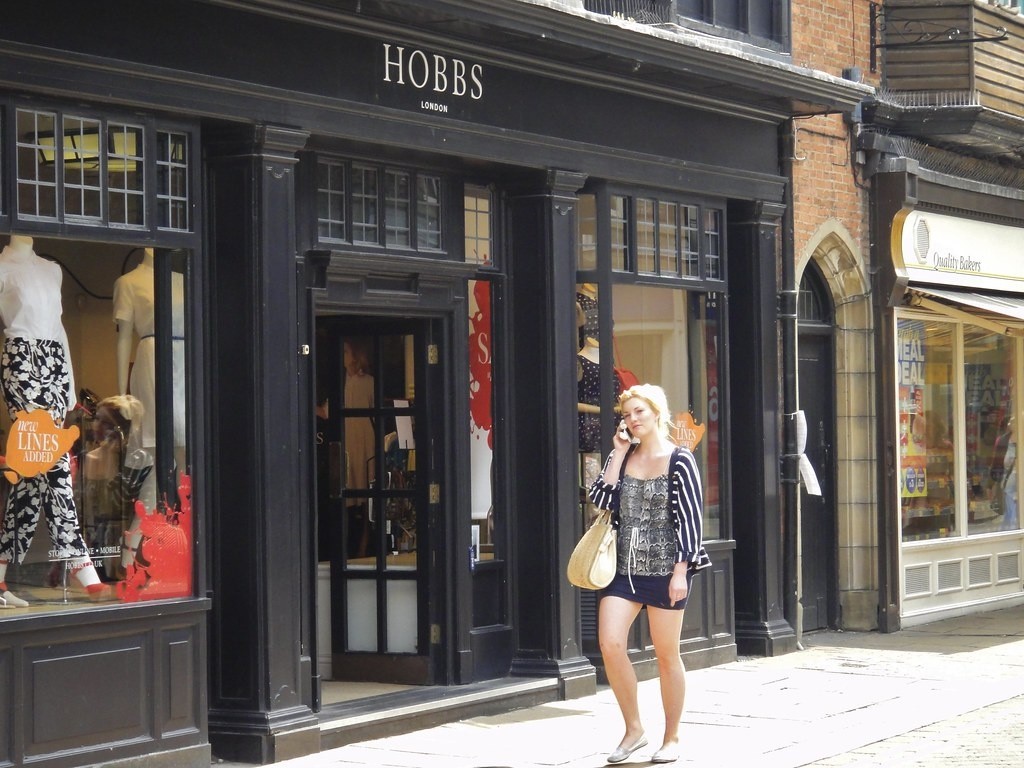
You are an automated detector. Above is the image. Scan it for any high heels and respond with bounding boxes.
[70,562,112,598]
[0,560,8,591]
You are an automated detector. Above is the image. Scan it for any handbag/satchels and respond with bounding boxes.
[614,367,640,393]
[990,489,1006,515]
[566,509,617,591]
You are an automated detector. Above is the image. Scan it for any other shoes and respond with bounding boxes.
[651,742,681,762]
[607,730,649,762]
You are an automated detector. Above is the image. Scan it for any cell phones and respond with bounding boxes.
[623,428,634,442]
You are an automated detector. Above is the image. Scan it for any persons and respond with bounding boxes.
[0,236,111,607]
[590,383,702,764]
[73,395,145,581]
[577,283,624,452]
[113,247,185,581]
